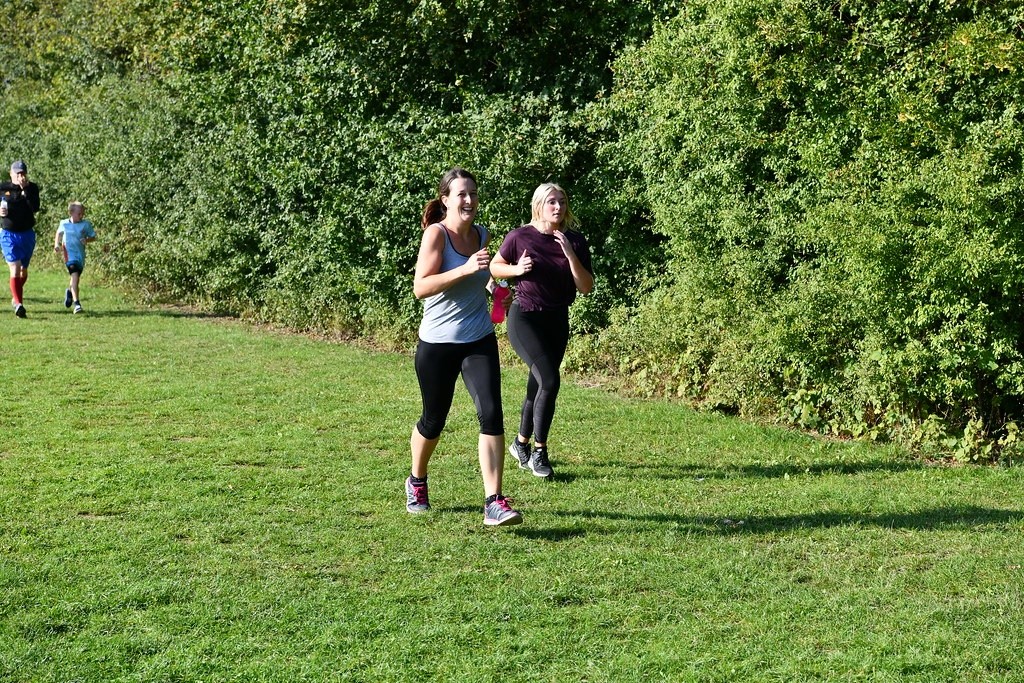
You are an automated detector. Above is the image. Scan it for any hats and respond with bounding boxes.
[12,161,27,172]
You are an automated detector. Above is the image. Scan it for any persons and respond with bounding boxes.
[404,169,523,525]
[54,201,97,314]
[0,161,40,318]
[489,184,596,476]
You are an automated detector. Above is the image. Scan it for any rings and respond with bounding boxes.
[483,260,487,265]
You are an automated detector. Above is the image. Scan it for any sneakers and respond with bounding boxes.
[509,436,531,469]
[528,450,554,477]
[405,476,431,514]
[483,494,523,526]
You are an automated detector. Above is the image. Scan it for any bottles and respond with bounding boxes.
[490,278,509,323]
[0,197,8,217]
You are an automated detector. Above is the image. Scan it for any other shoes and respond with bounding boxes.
[65,289,72,307]
[15,304,26,318]
[74,304,83,314]
[11,298,16,306]
